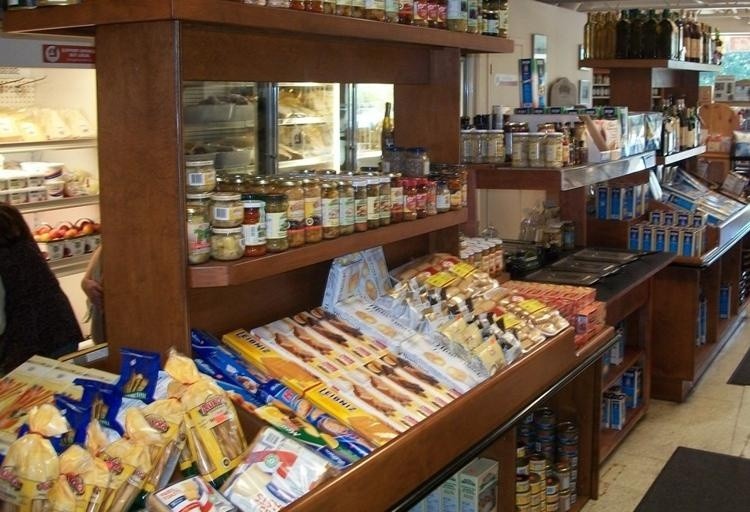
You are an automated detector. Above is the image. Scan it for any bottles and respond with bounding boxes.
[656,99,700,155]
[459,235,506,281]
[380,99,468,222]
[446,0,509,36]
[544,219,578,262]
[583,6,727,63]
[462,120,587,169]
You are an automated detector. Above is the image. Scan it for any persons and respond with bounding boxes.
[0,200,84,375]
[81,243,107,345]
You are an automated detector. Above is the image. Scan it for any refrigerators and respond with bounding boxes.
[1,33,106,361]
[184,81,354,182]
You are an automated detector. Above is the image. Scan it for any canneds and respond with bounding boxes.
[184,160,469,265]
[245,0,510,38]
[458,122,586,168]
[382,144,432,178]
[457,201,577,278]
[516,407,583,512]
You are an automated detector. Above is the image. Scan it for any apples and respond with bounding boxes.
[31,221,101,243]
